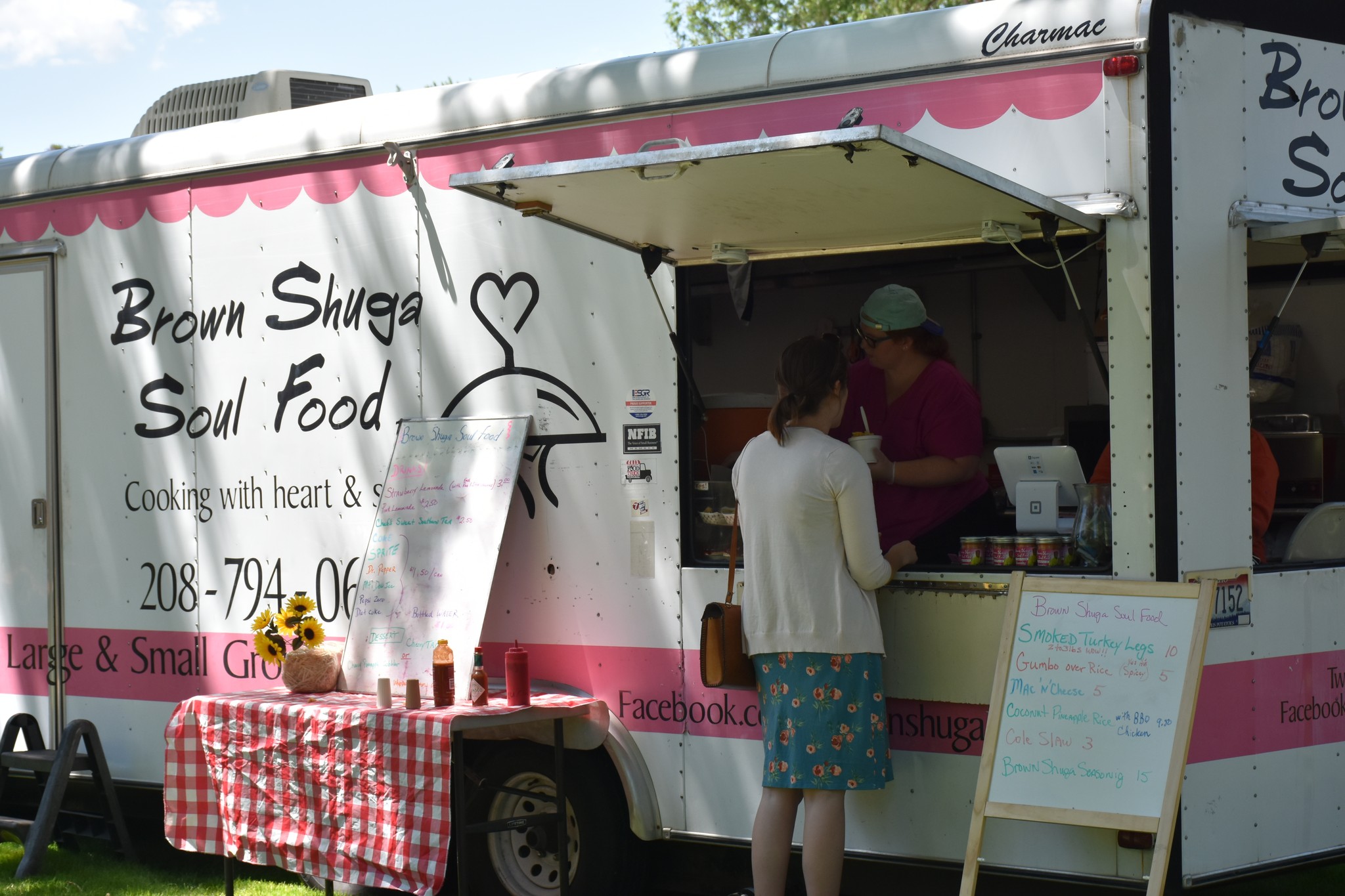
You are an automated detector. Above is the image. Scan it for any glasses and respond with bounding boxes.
[853,322,891,349]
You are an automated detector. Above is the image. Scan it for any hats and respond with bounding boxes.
[861,284,941,331]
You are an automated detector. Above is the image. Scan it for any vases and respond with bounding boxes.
[1071,483,1113,570]
[283,651,339,692]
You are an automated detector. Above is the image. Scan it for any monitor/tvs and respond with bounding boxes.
[994,445,1087,536]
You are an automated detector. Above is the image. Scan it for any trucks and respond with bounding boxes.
[0,0,1345,895]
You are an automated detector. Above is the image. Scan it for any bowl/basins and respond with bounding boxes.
[696,506,739,525]
[848,435,883,463]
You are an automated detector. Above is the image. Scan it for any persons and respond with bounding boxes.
[1087,428,1279,564]
[828,283,990,553]
[732,329,918,896]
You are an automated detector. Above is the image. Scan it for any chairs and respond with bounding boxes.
[1281,502,1345,563]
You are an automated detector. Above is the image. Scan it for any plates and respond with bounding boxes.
[705,554,727,561]
[723,555,744,561]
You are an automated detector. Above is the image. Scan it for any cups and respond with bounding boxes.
[376,678,392,708]
[405,679,421,710]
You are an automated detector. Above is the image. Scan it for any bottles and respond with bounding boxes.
[505,639,530,706]
[1071,483,1112,566]
[432,640,455,707]
[471,647,489,708]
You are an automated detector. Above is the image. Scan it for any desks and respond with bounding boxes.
[165,688,611,896]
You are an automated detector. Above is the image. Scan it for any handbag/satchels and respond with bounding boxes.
[698,603,757,691]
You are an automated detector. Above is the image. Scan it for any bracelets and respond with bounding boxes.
[888,461,897,484]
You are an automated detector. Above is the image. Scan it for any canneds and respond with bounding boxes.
[959,534,1074,565]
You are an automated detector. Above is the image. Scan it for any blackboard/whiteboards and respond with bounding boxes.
[335,415,534,698]
[980,569,1217,832]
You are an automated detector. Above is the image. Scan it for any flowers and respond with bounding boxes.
[251,594,327,666]
